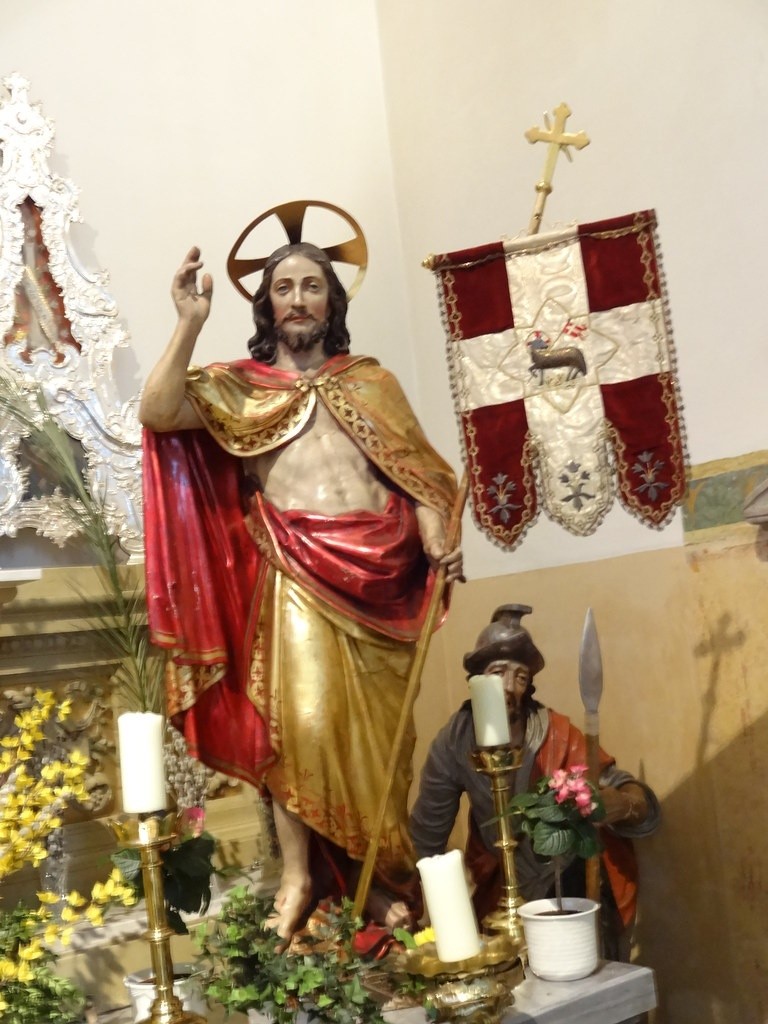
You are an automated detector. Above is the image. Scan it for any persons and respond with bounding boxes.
[136,198,471,959]
[408,602,664,961]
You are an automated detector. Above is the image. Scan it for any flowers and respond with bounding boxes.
[508,763,608,913]
[0,688,221,1024]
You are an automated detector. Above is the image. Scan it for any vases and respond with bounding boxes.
[518,897,602,983]
[123,962,212,1024]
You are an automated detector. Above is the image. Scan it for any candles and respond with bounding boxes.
[468,674,510,747]
[416,848,482,964]
[118,710,167,814]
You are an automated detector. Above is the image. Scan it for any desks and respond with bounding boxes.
[382,959,658,1024]
